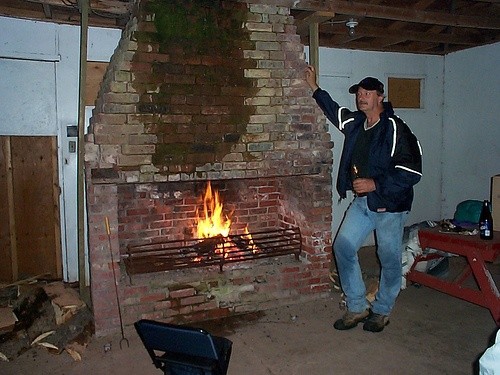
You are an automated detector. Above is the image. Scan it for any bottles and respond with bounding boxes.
[479,200,493,240]
[352,164,368,197]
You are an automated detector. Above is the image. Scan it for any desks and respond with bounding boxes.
[407,224,500,326]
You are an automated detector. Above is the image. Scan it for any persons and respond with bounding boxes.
[305,64,423,332]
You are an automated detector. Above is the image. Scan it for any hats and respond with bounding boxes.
[348,77,385,94]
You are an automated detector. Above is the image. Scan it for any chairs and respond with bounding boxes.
[135,319,233,375]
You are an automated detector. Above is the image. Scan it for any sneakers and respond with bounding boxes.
[363,313,390,332]
[334,309,369,330]
